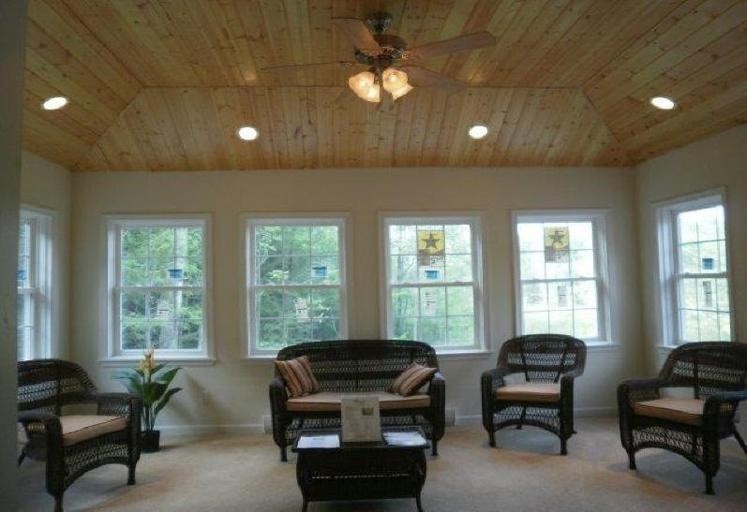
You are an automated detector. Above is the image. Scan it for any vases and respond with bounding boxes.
[140,429,161,452]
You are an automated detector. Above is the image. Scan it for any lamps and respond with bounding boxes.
[346,54,415,103]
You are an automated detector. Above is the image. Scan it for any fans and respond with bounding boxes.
[260,11,499,110]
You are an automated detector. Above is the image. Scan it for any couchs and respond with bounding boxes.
[266,338,447,463]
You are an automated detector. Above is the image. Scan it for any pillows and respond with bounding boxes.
[273,354,321,400]
[386,360,438,398]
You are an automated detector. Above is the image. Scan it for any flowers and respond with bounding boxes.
[108,347,186,432]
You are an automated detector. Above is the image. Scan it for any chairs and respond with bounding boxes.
[617,341,747,496]
[17,359,143,512]
[480,333,587,456]
